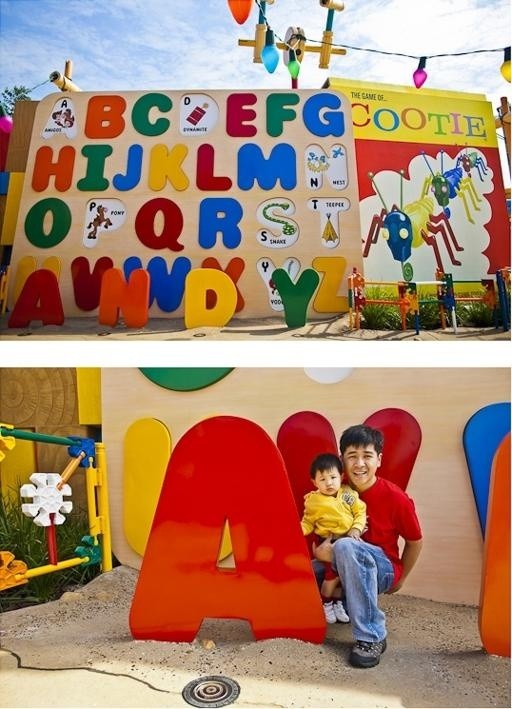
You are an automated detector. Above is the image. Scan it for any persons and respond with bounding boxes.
[299,453,367,625]
[309,424,423,669]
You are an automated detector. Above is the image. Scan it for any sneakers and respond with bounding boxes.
[349,638,387,667]
[323,600,349,624]
